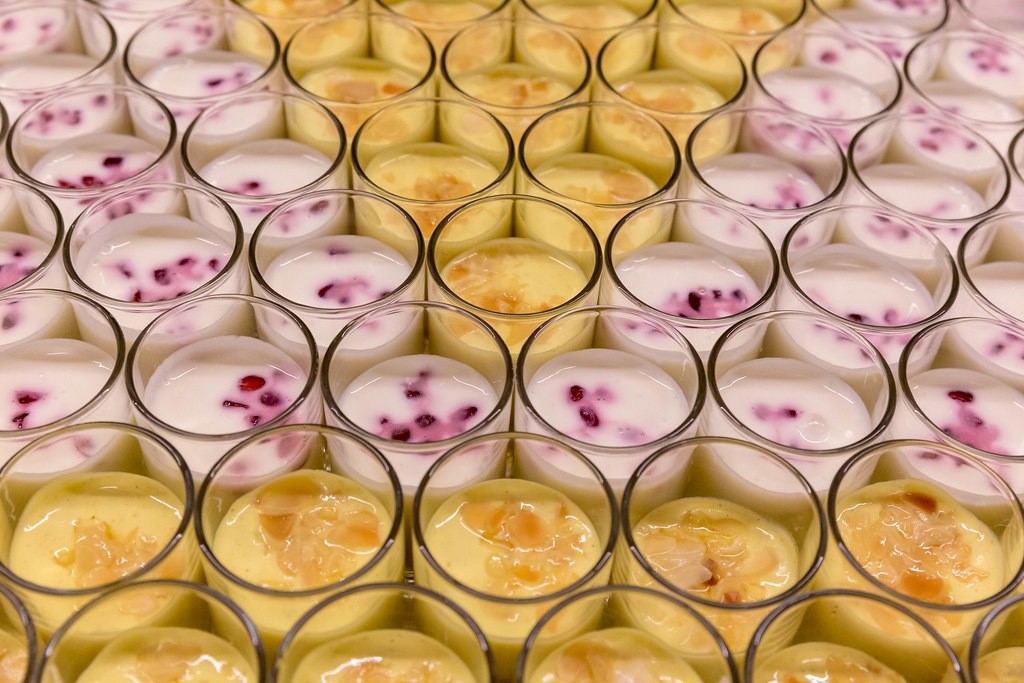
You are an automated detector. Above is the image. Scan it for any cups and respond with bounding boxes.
[1,0,1024,683]
